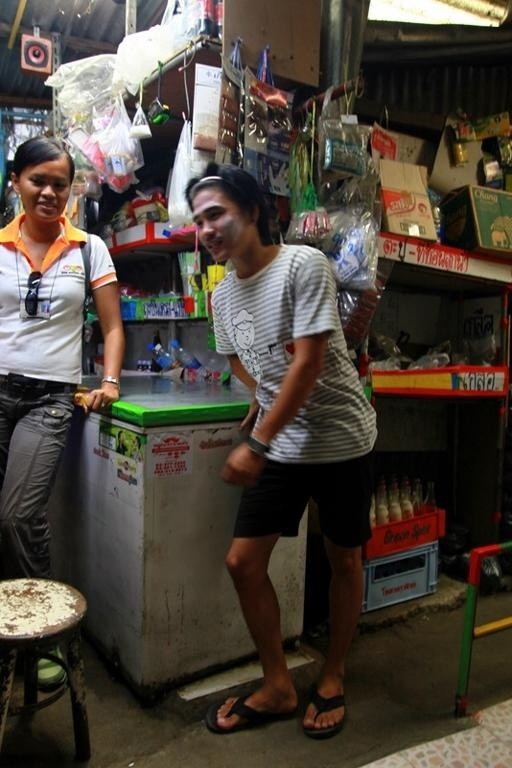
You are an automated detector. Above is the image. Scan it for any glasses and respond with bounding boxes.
[25,271,42,316]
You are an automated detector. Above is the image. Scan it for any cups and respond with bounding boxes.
[207,265,226,291]
[188,291,206,318]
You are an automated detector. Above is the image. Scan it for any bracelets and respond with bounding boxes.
[245,435,271,455]
[101,376,120,385]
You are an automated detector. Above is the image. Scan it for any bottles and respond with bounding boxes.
[149,106,181,149]
[136,333,231,385]
[366,472,433,526]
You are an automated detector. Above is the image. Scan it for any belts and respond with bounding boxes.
[0,372,78,394]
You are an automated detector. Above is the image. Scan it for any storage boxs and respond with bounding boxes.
[362,122,426,162]
[377,159,439,242]
[441,183,512,255]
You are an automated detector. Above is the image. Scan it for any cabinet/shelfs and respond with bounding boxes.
[346,231,512,407]
[102,235,211,323]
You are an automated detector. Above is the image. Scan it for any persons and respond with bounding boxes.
[184,161,380,738]
[1,134,126,691]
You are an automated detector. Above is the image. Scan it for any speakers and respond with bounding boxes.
[21,34,52,75]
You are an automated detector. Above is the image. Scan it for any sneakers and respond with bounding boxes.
[37,645,67,693]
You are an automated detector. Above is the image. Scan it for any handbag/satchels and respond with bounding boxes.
[79,232,100,379]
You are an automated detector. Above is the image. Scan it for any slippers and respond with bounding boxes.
[205,685,303,735]
[301,682,346,741]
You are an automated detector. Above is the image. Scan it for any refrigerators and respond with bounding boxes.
[43,374,312,699]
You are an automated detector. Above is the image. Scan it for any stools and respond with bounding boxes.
[0,576,92,767]
